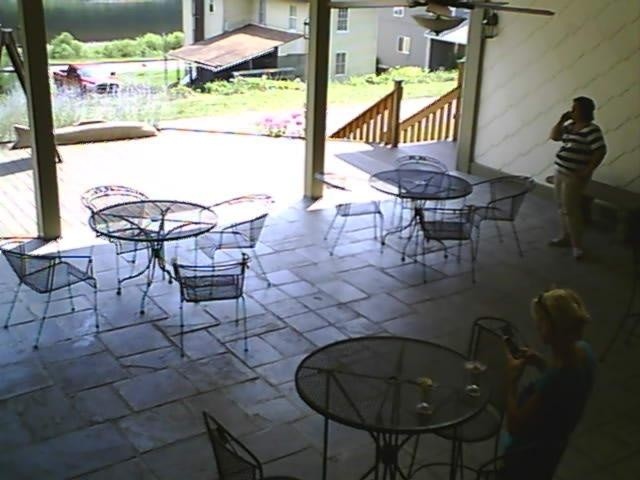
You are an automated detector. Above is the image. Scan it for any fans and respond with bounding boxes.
[326,0,557,20]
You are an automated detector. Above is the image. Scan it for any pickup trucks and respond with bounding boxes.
[53,62,121,96]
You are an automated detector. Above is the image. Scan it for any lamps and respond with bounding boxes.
[481,9,501,39]
[412,0,469,36]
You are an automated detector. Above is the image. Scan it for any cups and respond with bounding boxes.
[415,377,434,414]
[466,360,482,401]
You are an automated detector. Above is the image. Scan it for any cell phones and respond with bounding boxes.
[502,335,523,360]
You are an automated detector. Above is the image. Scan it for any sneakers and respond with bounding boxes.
[550,238,583,257]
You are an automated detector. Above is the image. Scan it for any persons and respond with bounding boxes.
[494,288,597,480]
[549,95,606,260]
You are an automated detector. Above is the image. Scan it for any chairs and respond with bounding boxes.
[314,154,535,284]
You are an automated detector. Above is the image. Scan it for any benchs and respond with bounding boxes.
[546,174,640,244]
